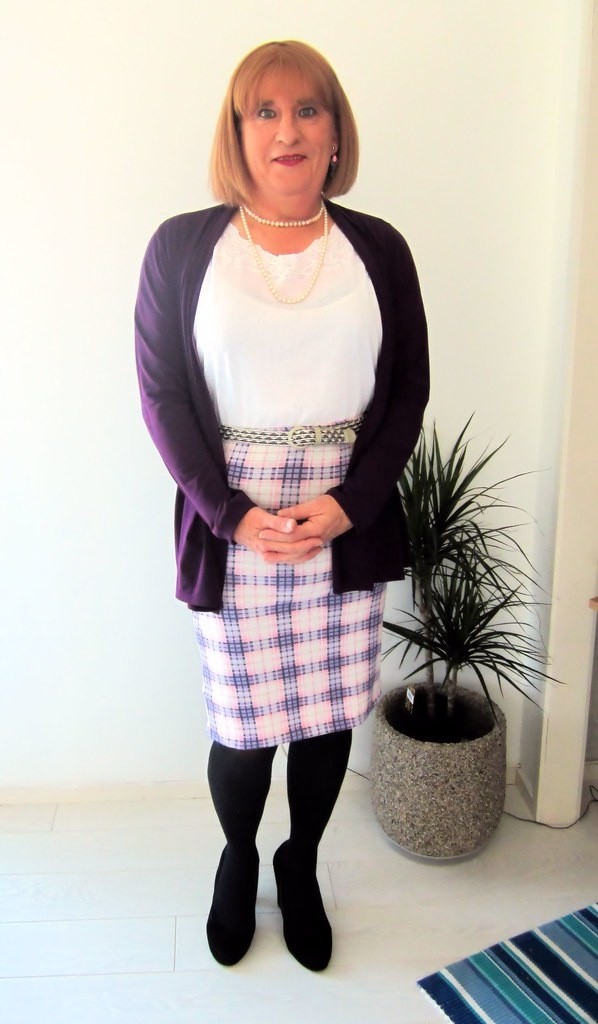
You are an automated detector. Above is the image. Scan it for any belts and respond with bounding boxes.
[218,417,364,449]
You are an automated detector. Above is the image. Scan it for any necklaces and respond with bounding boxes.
[238,201,329,304]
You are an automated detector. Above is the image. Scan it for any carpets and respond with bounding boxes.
[415,900,598,1024]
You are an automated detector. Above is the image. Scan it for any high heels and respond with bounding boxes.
[207,841,259,964]
[272,838,333,971]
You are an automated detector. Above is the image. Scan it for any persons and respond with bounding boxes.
[133,42,430,971]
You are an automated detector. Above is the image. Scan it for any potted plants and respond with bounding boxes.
[368,409,569,868]
